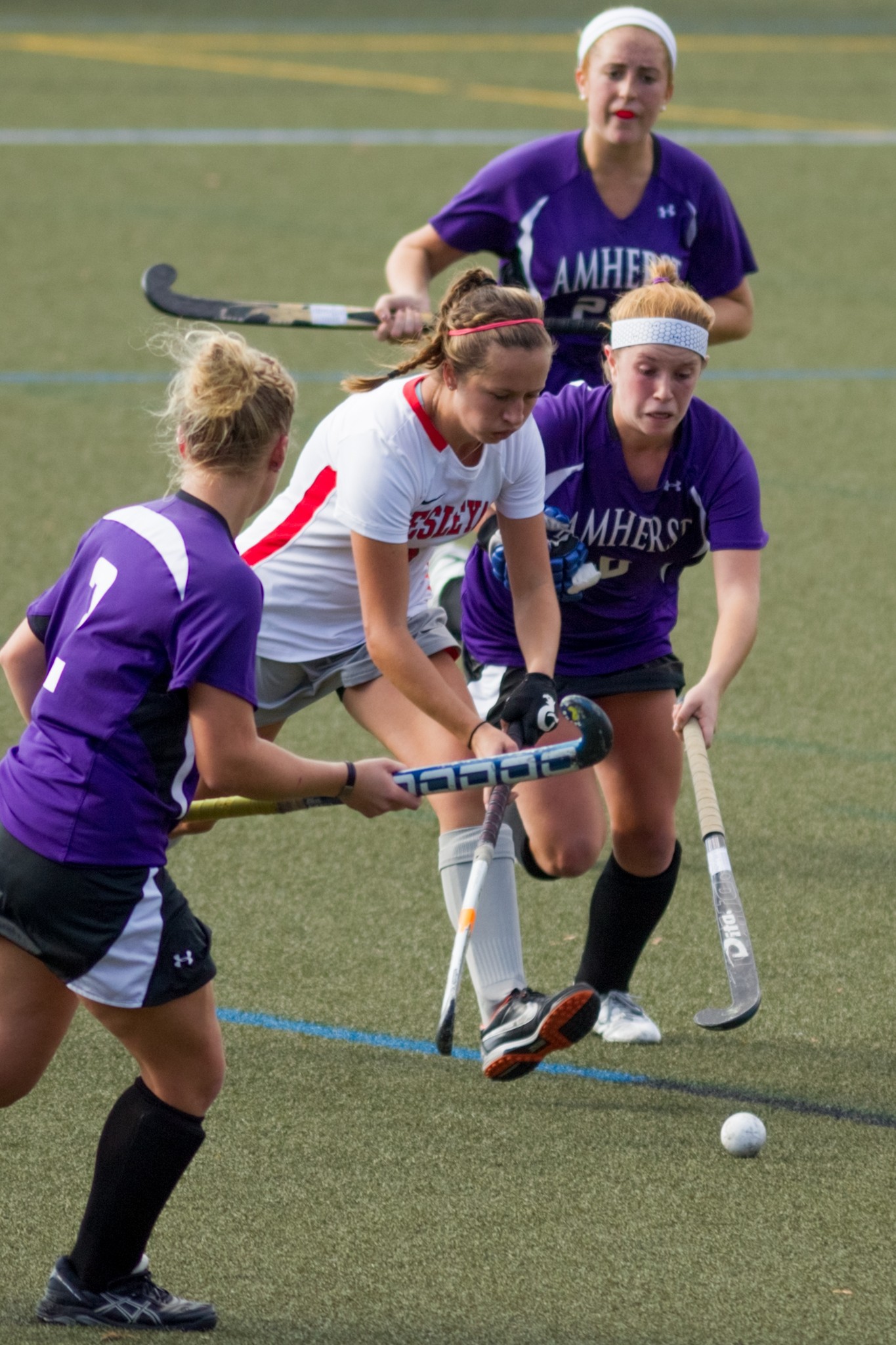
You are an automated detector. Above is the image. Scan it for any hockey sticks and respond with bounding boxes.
[679,718,761,1031]
[180,692,613,824]
[140,263,609,336]
[435,723,524,1056]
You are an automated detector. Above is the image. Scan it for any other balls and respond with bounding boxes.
[720,1112,766,1157]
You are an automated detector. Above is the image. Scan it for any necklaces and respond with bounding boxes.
[429,382,483,462]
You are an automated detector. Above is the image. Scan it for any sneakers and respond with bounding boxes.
[35,1254,218,1331]
[590,989,662,1045]
[477,983,600,1081]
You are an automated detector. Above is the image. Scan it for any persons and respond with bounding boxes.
[0,317,421,1334]
[373,4,760,394]
[459,253,768,1048]
[161,265,602,1085]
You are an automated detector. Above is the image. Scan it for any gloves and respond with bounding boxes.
[496,671,561,746]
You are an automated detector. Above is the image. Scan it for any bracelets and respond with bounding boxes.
[339,761,357,804]
[467,720,489,748]
